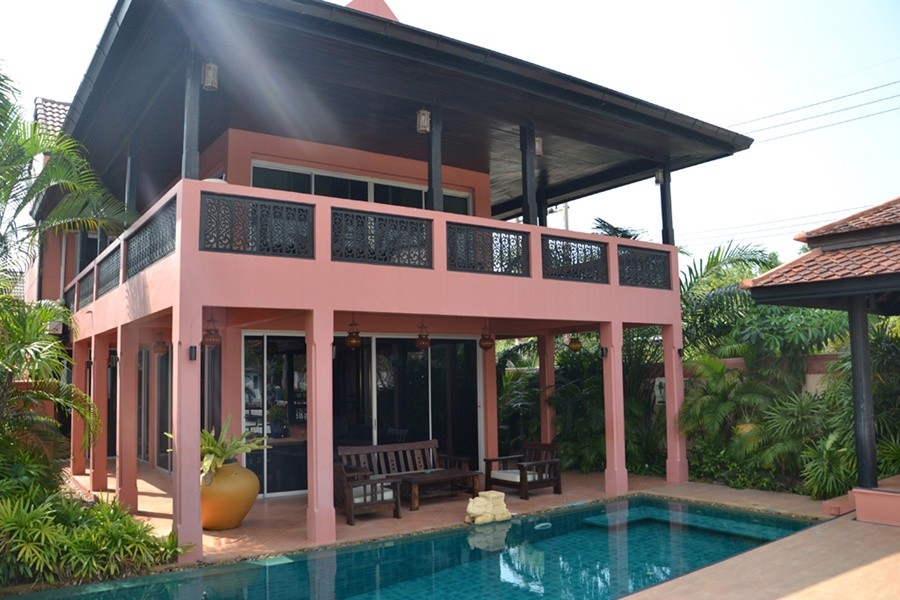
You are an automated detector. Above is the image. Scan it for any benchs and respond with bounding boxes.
[337,437,472,503]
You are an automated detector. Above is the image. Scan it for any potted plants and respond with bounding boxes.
[164,416,272,530]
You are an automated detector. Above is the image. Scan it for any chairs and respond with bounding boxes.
[483,441,562,500]
[333,462,403,526]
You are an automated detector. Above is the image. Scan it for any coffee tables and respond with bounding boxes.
[387,466,481,510]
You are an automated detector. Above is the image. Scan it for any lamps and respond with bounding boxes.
[678,349,684,357]
[190,345,197,360]
[535,135,543,158]
[601,347,608,357]
[202,60,218,91]
[346,312,362,350]
[416,314,430,350]
[155,329,165,354]
[332,345,336,358]
[655,168,664,184]
[479,318,494,351]
[416,104,430,134]
[569,330,581,354]
[203,308,221,349]
